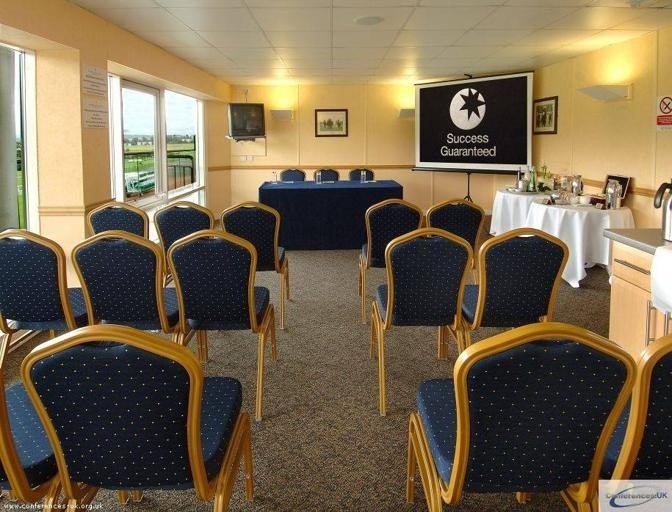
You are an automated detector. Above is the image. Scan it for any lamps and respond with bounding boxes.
[395,108,415,122]
[270,108,295,123]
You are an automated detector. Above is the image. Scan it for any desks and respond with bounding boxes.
[486,184,565,243]
[256,179,404,253]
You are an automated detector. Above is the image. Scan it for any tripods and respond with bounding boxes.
[463,172,490,235]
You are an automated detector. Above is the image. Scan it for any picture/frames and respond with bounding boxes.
[314,108,349,137]
[531,95,558,136]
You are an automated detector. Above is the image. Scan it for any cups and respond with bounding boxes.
[571,196,592,206]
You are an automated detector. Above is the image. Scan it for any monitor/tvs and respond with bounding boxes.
[227,102,265,137]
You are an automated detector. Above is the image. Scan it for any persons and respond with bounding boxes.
[536,111,552,127]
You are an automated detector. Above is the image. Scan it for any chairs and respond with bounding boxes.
[312,168,340,182]
[352,195,672,511]
[1,194,292,510]
[348,168,375,182]
[278,167,307,182]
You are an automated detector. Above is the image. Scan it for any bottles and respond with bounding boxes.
[316,171,322,181]
[360,171,366,183]
[515,167,538,193]
[562,173,582,193]
[605,178,622,210]
